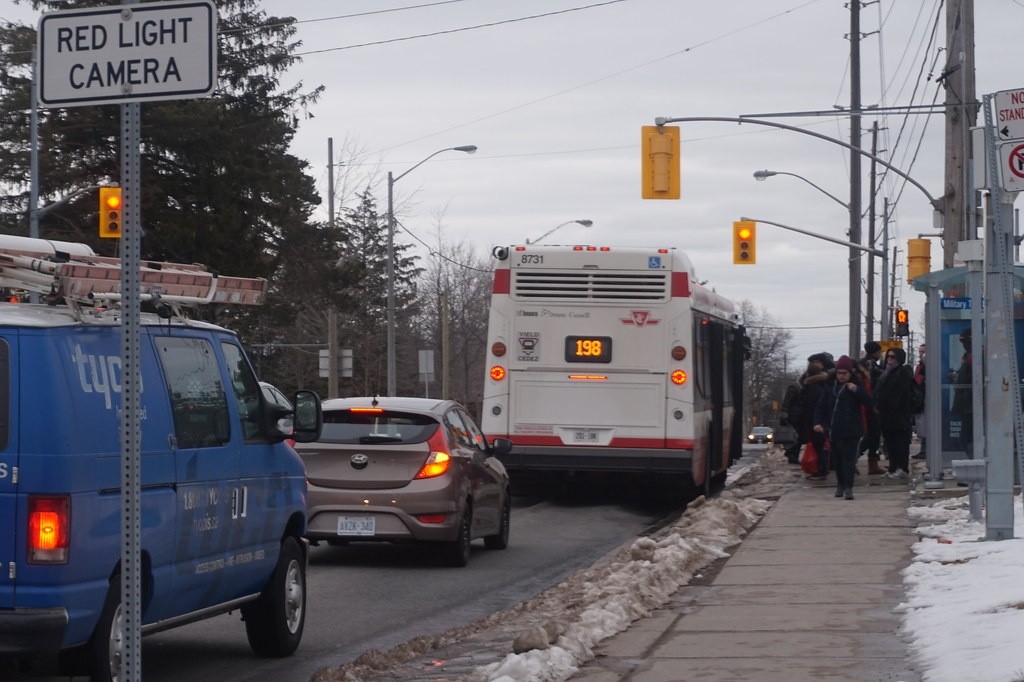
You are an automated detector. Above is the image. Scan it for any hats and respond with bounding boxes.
[833,355,855,374]
[865,341,882,354]
[808,353,826,363]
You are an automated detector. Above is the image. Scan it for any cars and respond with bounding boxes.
[281,396,515,567]
[748,426,774,444]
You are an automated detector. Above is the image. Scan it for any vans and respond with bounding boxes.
[0,301,325,682]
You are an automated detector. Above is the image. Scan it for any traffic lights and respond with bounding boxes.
[732,220,756,263]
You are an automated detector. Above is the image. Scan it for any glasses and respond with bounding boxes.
[887,355,896,359]
[959,335,972,344]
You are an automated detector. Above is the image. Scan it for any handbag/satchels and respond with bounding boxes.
[772,422,799,445]
[801,432,819,475]
[798,444,806,465]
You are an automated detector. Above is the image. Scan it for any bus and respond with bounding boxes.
[478,244,756,512]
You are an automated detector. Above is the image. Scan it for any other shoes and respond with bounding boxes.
[911,451,926,459]
[866,462,888,476]
[882,471,893,479]
[854,465,860,477]
[896,470,908,479]
[783,450,801,465]
[843,488,854,500]
[806,472,827,481]
[835,487,844,497]
[956,480,969,488]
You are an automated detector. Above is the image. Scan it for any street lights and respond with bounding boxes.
[386,143,479,397]
[752,170,863,367]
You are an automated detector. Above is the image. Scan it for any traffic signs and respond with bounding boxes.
[994,86,1024,144]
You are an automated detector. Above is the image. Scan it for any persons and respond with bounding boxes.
[856,341,887,475]
[779,371,810,465]
[799,353,837,480]
[948,326,974,459]
[813,355,873,499]
[912,343,927,459]
[873,347,914,480]
